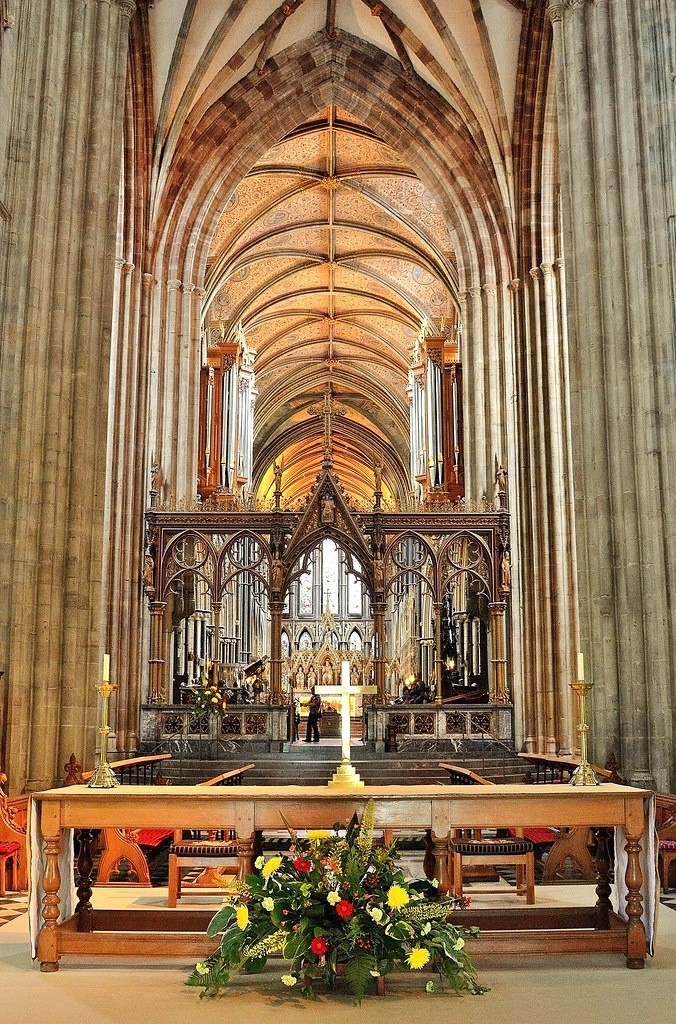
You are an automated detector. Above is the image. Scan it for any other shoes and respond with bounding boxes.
[302,739,311,742]
[313,739,319,742]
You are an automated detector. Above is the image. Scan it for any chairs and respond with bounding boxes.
[434,779,536,904]
[168,825,255,907]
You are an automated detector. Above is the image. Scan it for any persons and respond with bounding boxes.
[300,685,321,743]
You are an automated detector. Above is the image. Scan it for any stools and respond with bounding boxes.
[0,842,20,896]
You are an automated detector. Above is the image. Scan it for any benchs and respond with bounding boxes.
[439,761,561,859]
[74,755,177,888]
[505,753,612,885]
[654,794,676,894]
[0,773,30,891]
[121,761,258,882]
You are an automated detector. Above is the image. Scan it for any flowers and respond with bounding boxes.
[183,801,486,1009]
[187,672,231,719]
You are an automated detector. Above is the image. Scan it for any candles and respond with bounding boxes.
[576,651,585,681]
[103,653,110,681]
